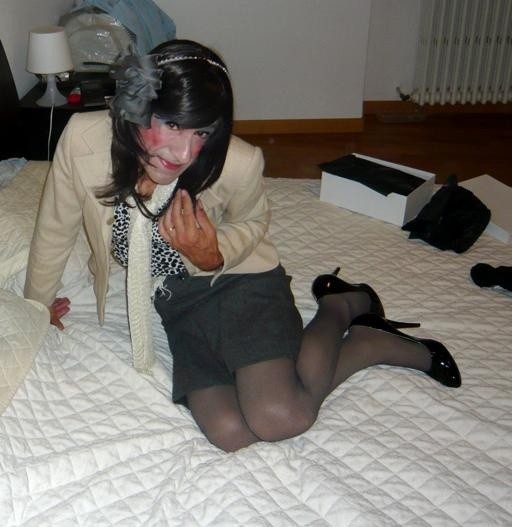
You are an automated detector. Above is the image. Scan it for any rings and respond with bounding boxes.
[167,227,174,233]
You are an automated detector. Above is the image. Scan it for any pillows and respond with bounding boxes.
[0,290,51,414]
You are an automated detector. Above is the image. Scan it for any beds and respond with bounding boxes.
[0,159,512,527]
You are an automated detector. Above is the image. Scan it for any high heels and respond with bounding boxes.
[348,315,463,391]
[310,266,390,317]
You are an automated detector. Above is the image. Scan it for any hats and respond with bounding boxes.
[64,11,133,74]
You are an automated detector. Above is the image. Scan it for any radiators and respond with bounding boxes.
[396,0,511,106]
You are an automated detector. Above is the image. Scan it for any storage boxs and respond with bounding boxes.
[319,151,437,228]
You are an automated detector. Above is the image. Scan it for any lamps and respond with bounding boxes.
[24,26,74,108]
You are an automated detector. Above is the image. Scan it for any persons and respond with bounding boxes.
[22,39,462,453]
